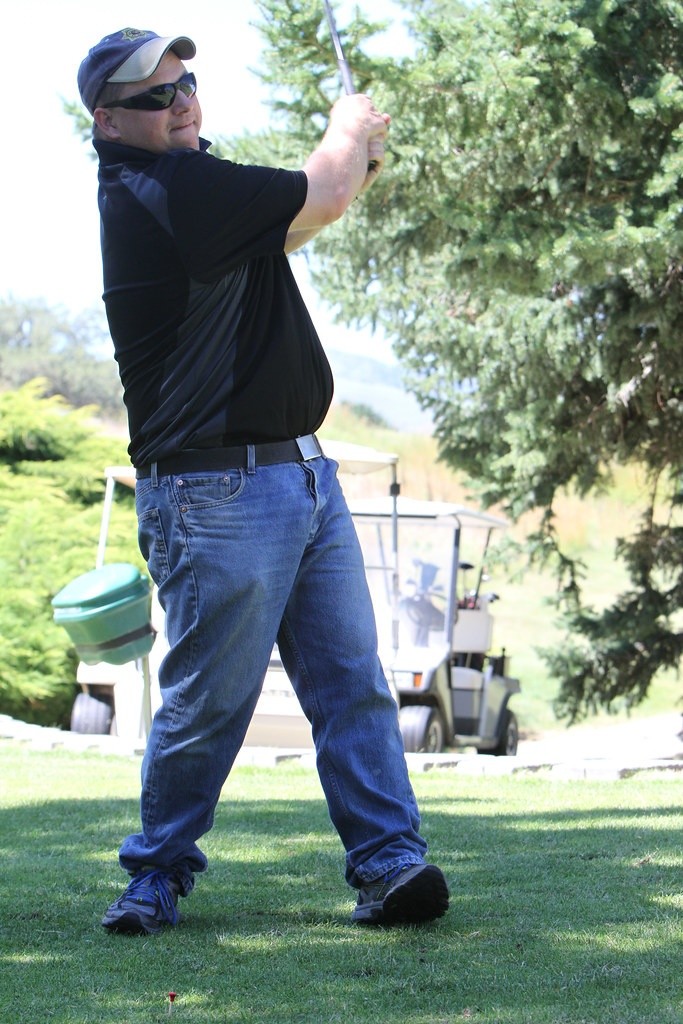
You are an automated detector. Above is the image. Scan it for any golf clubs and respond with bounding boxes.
[456,560,503,612]
[320,0,378,171]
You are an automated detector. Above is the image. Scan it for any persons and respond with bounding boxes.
[73,30,449,931]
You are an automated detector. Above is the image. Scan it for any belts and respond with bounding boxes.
[135,433,324,480]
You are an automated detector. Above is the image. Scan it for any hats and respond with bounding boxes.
[77,27,196,116]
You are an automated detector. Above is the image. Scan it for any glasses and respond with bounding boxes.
[98,72,197,110]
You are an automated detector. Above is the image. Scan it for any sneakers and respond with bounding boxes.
[351,863,449,928]
[101,865,182,934]
